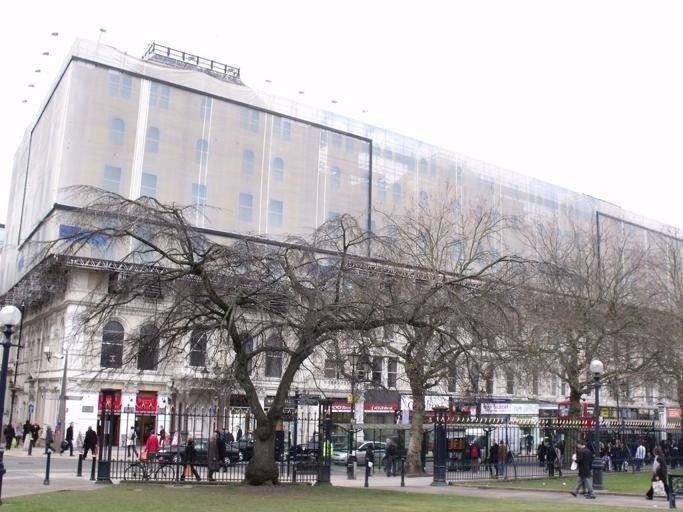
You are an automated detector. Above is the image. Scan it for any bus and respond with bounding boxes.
[557,400,659,466]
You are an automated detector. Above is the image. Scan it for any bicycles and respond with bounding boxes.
[123,458,175,482]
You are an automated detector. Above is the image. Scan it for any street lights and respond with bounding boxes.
[589,359,604,490]
[212,362,222,438]
[0,305,24,506]
[347,345,360,479]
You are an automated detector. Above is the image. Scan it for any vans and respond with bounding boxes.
[451,424,521,464]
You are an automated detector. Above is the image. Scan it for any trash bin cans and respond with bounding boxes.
[347,456,357,478]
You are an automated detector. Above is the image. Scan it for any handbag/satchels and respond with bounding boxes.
[61,440,69,450]
[652,480,667,497]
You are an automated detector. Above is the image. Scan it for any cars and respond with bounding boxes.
[295,441,388,466]
[156,439,254,467]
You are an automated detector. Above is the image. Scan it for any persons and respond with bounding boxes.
[127,425,242,481]
[528,431,682,501]
[323,439,333,464]
[464,440,507,476]
[3,420,97,460]
[365,437,400,477]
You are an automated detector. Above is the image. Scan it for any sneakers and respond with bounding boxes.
[570,490,595,499]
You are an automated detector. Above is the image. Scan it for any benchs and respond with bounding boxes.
[667,474,683,508]
